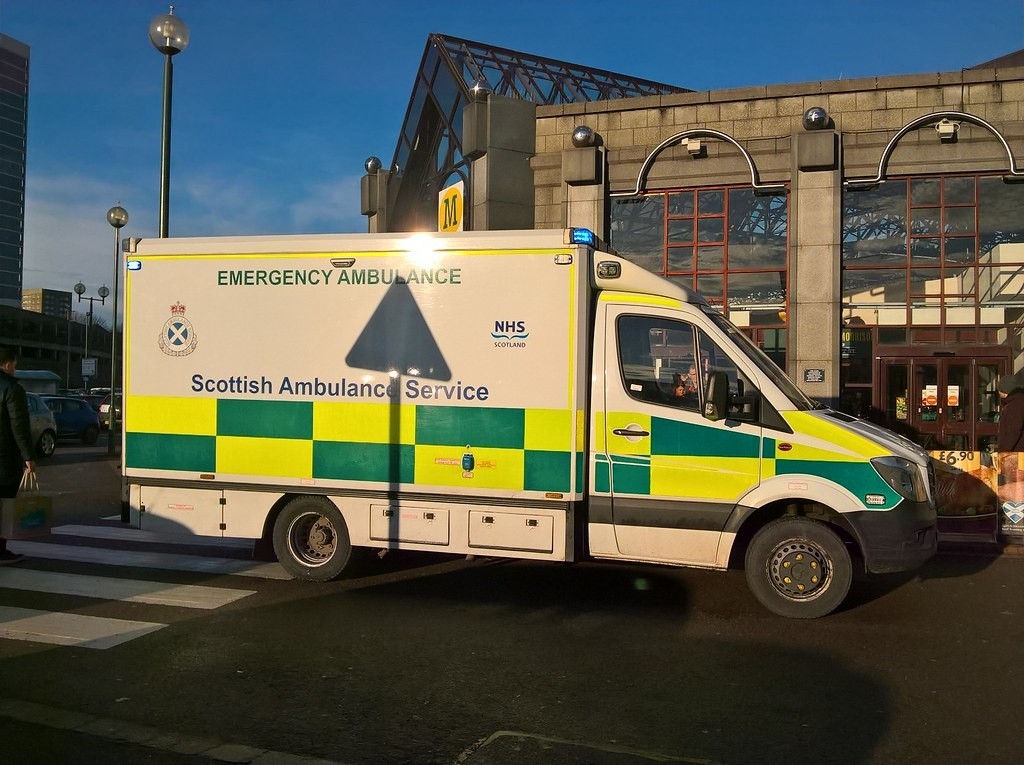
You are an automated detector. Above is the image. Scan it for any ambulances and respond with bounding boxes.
[118,227,940,620]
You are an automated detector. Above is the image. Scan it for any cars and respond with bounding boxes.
[97,392,122,430]
[25,392,57,459]
[28,397,100,446]
[68,395,106,413]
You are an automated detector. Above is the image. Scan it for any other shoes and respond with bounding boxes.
[0,550,24,564]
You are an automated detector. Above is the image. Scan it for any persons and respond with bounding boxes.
[0,345,36,563]
[996,375,1024,453]
[958,409,963,420]
[674,365,697,396]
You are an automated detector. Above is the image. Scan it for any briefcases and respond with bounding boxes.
[0,469,56,540]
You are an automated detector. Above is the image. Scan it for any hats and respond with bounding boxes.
[676,379,685,387]
[998,375,1019,394]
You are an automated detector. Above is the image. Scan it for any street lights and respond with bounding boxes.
[148,5,190,238]
[74,281,110,359]
